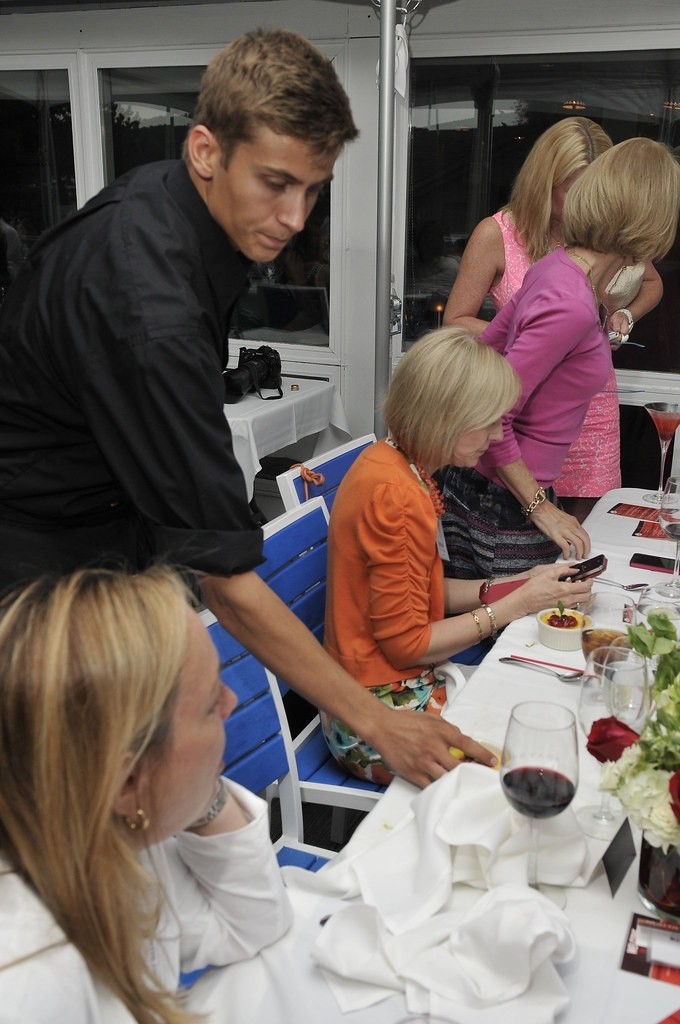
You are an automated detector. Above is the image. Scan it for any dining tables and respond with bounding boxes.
[199,489,680,1024]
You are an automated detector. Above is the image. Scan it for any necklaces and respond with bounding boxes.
[568,248,608,332]
[393,437,446,518]
[504,207,562,255]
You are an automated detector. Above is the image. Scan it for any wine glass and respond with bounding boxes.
[642,401,680,504]
[499,701,579,909]
[577,647,648,840]
[580,592,636,706]
[632,586,680,703]
[653,476,680,598]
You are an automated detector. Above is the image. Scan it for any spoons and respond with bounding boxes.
[592,575,650,591]
[499,657,583,682]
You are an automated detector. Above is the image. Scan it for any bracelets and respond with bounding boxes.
[613,309,634,333]
[521,486,546,517]
[471,611,483,645]
[187,777,227,829]
[482,604,497,637]
[479,578,495,605]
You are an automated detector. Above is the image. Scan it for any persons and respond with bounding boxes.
[442,117,663,525]
[0,217,24,304]
[321,327,592,785]
[429,138,680,651]
[0,567,293,1023]
[0,27,497,790]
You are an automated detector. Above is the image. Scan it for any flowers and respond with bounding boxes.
[579,609,680,848]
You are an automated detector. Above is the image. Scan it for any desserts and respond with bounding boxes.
[539,600,590,631]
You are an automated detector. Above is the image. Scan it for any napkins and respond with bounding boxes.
[314,760,588,1024]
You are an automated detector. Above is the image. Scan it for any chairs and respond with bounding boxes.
[275,432,380,591]
[255,496,391,838]
[181,610,339,991]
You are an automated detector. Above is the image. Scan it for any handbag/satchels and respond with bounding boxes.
[603,258,646,318]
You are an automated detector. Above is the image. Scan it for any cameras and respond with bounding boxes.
[222,345,282,405]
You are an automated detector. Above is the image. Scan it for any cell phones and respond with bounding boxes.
[630,553,680,575]
[569,555,607,582]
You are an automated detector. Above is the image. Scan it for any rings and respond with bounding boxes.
[577,603,580,610]
